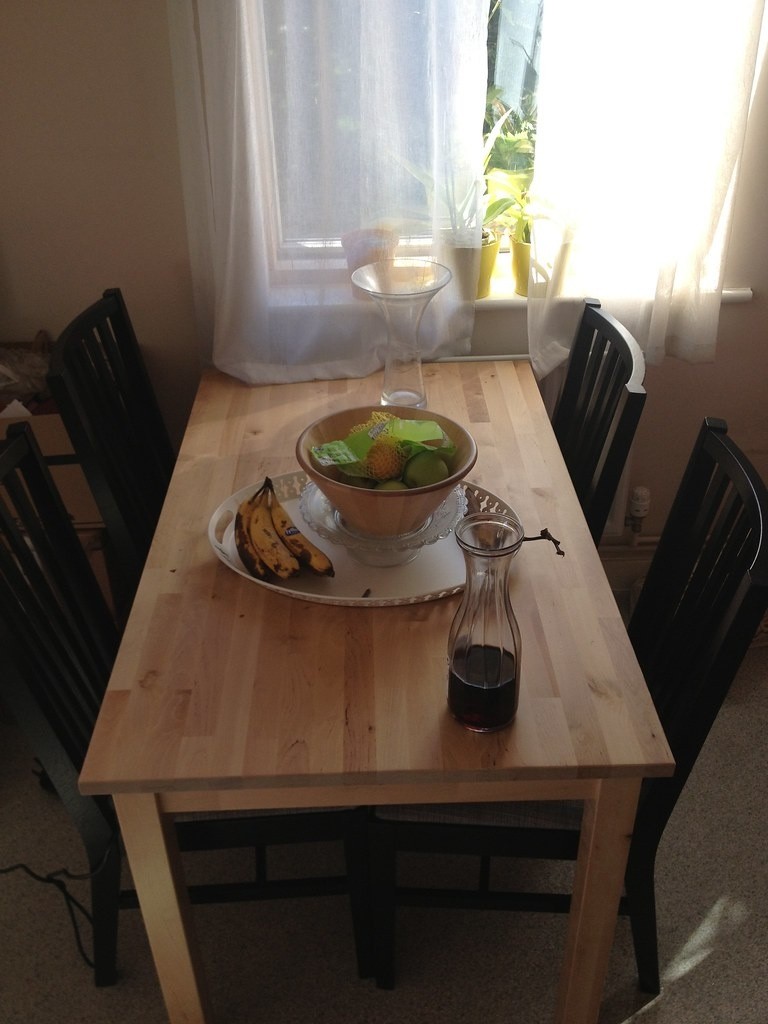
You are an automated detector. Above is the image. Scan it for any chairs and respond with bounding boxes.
[0,420,372,985]
[550,296,646,550]
[371,414,768,993]
[46,287,178,603]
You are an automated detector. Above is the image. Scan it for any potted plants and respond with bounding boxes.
[381,89,570,298]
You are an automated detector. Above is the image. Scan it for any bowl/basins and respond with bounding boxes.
[295,404,477,537]
[299,480,468,568]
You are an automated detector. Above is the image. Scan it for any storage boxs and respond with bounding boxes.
[0,397,134,634]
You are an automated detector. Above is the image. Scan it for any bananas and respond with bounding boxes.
[234,477,335,585]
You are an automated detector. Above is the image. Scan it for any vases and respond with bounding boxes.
[342,229,399,299]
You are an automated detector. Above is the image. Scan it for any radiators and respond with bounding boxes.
[434,353,650,549]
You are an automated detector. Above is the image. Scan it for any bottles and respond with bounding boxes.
[447,512,524,734]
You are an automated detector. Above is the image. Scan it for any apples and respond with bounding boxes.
[342,451,448,490]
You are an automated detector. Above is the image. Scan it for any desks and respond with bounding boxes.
[77,364,676,1024]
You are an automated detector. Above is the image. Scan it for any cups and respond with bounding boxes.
[341,229,398,300]
[351,261,452,410]
